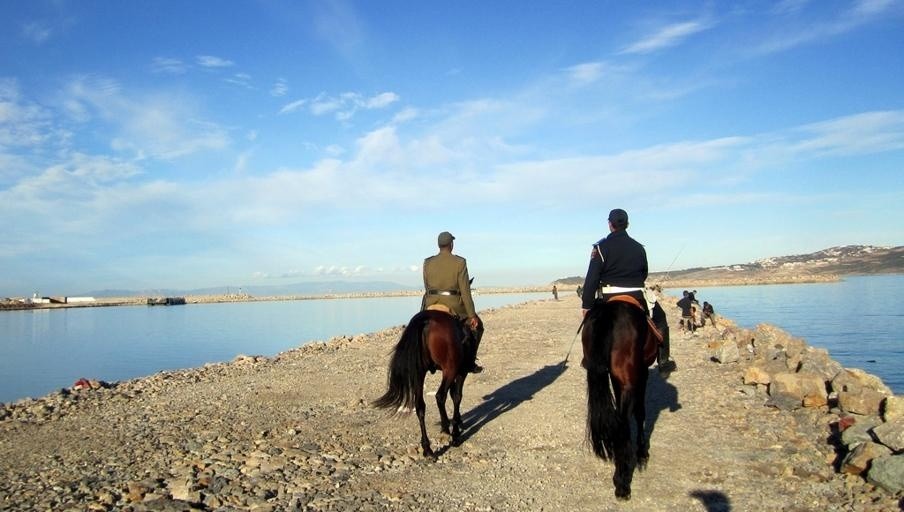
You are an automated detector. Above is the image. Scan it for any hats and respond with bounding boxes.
[609,209,628,223]
[438,232,455,246]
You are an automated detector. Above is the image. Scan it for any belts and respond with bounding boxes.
[429,290,457,295]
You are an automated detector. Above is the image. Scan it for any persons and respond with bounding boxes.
[579,208,677,375]
[422,231,484,374]
[575,285,581,297]
[674,289,718,333]
[552,285,557,299]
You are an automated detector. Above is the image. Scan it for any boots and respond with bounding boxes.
[657,326,676,372]
[469,328,484,372]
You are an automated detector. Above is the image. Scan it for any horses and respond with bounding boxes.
[371,277,476,463]
[579,299,660,503]
